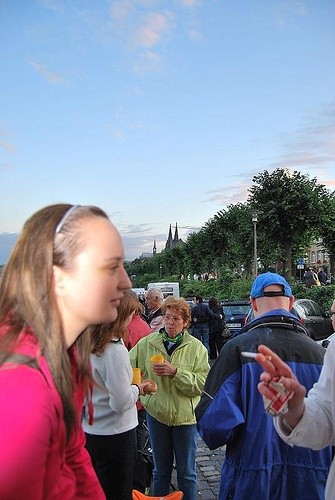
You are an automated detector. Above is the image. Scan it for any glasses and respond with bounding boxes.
[329,312,335,317]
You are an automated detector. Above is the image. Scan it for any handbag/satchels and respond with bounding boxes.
[221,321,232,338]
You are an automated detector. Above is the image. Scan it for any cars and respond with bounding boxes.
[187,298,334,341]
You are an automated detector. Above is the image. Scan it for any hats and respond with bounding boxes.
[249,271,292,299]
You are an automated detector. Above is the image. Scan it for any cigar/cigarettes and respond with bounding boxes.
[241,352,272,360]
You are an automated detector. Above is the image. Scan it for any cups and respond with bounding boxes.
[151,355,164,363]
[132,368,140,384]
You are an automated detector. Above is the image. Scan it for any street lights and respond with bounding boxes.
[249,207,264,279]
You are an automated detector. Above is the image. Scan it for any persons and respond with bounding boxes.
[255,298,335,500]
[145,288,165,333]
[0,204,132,500]
[81,290,151,500]
[188,295,222,360]
[122,301,153,497]
[193,297,225,360]
[128,296,211,500]
[303,267,330,289]
[194,272,335,500]
